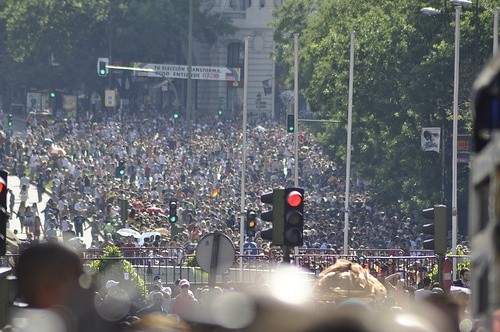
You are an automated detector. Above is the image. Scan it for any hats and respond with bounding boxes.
[105,280,119,289]
[154,276,161,281]
[179,279,190,287]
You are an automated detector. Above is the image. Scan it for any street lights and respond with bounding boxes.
[420,6,456,18]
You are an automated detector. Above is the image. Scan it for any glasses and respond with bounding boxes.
[180,285,189,288]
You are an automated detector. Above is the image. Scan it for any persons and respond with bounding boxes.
[0,79,500,332]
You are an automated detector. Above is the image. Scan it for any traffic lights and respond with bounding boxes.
[168,200,178,223]
[99,61,107,75]
[246,208,256,237]
[274,187,305,246]
[287,115,294,133]
[260,188,275,246]
